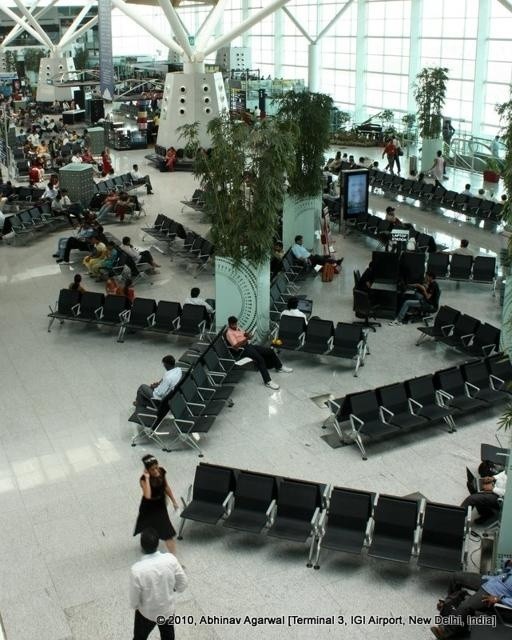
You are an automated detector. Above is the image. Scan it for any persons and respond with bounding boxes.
[291,235,344,266]
[460,476,507,525]
[280,298,308,326]
[254,105,262,120]
[0,92,161,300]
[388,270,442,326]
[320,120,507,234]
[133,454,186,569]
[132,355,183,407]
[130,527,189,640]
[248,74,283,80]
[225,315,294,390]
[431,557,512,640]
[182,287,215,314]
[164,146,177,171]
[436,239,473,256]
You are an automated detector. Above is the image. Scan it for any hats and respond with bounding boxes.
[482,477,496,484]
[386,206,395,213]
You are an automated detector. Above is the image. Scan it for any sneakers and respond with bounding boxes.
[430,626,445,640]
[265,379,280,390]
[276,364,293,373]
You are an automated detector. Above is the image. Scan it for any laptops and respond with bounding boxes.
[466,466,494,494]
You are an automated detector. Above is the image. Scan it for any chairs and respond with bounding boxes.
[0,100,512,640]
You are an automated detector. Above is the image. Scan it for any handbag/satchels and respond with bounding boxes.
[441,590,468,617]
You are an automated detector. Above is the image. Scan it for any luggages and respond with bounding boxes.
[322,262,334,282]
